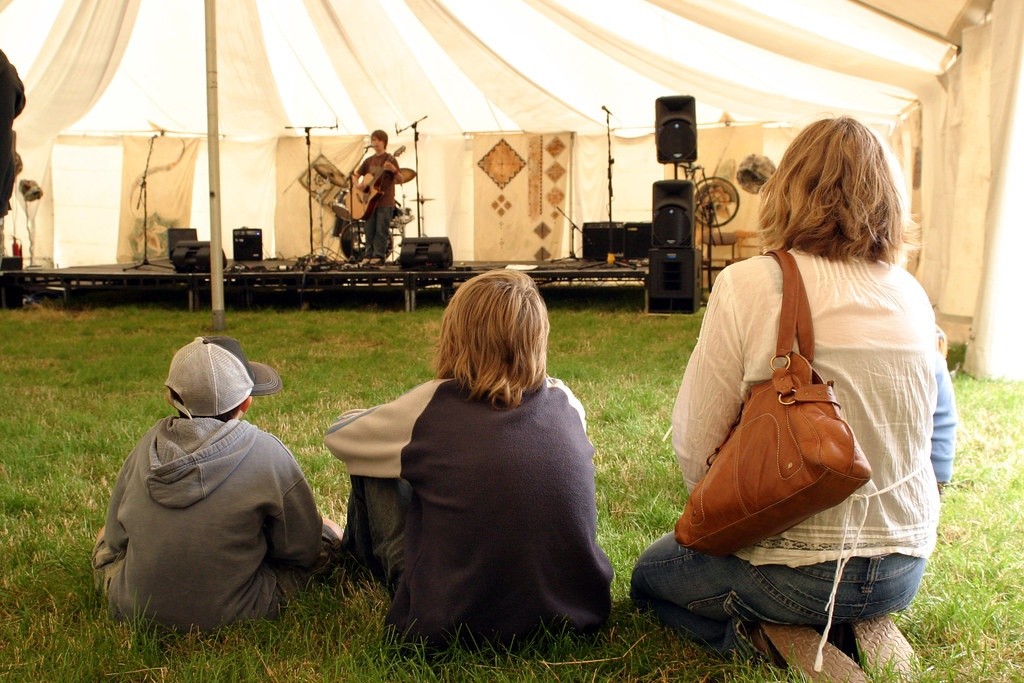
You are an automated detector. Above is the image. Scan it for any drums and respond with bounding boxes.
[339,220,394,262]
[331,189,350,214]
[389,207,415,228]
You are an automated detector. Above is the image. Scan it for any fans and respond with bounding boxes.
[694,178,740,305]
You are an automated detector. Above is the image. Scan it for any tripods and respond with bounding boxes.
[333,147,382,271]
[123,138,176,272]
[551,206,593,262]
[578,115,637,271]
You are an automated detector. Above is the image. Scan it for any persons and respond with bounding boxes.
[91,333,344,627]
[627,117,959,683]
[353,130,404,268]
[0,49,27,217]
[324,269,614,666]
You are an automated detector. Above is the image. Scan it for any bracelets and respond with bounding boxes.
[393,170,398,175]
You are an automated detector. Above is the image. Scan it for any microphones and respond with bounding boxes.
[586,242,597,247]
[364,144,375,149]
[336,117,338,131]
[602,106,613,115]
[149,135,159,140]
[395,124,399,137]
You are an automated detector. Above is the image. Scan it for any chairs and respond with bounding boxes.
[735,232,763,261]
[703,233,738,269]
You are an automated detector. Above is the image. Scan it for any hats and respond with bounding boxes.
[165,336,284,419]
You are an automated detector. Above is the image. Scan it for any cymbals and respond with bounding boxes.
[411,198,434,202]
[313,163,349,188]
[393,168,416,185]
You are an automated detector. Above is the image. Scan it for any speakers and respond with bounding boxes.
[0,256,23,310]
[172,240,227,273]
[582,223,626,260]
[651,181,696,249]
[655,95,697,164]
[648,247,701,314]
[623,223,652,261]
[400,236,453,271]
[233,228,263,261]
[168,228,198,260]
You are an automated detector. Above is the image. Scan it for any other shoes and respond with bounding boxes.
[754,619,874,683]
[814,613,920,683]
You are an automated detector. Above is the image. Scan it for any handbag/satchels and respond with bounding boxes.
[674,249,872,556]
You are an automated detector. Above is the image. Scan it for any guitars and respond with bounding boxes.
[346,145,407,221]
